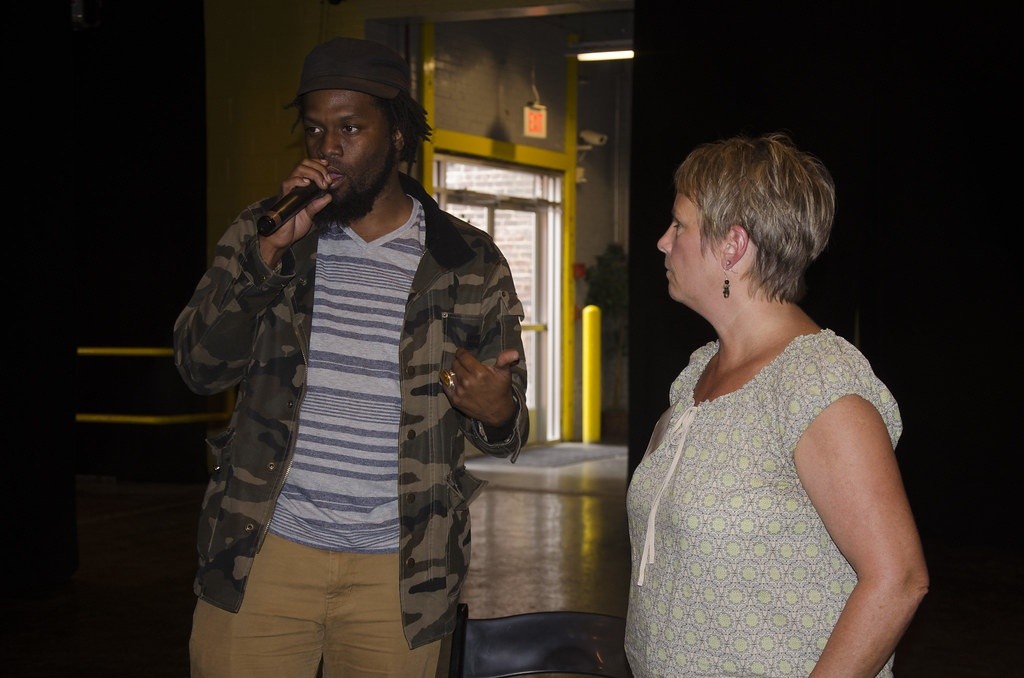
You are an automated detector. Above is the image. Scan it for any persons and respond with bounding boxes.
[625,138,932,677]
[175,37,530,678]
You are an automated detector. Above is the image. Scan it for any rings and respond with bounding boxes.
[439,370,456,390]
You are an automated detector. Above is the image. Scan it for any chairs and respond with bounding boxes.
[448,603,635,678]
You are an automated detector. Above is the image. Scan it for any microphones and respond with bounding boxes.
[257,181,329,235]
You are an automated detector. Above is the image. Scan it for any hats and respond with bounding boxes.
[285,37,408,105]
